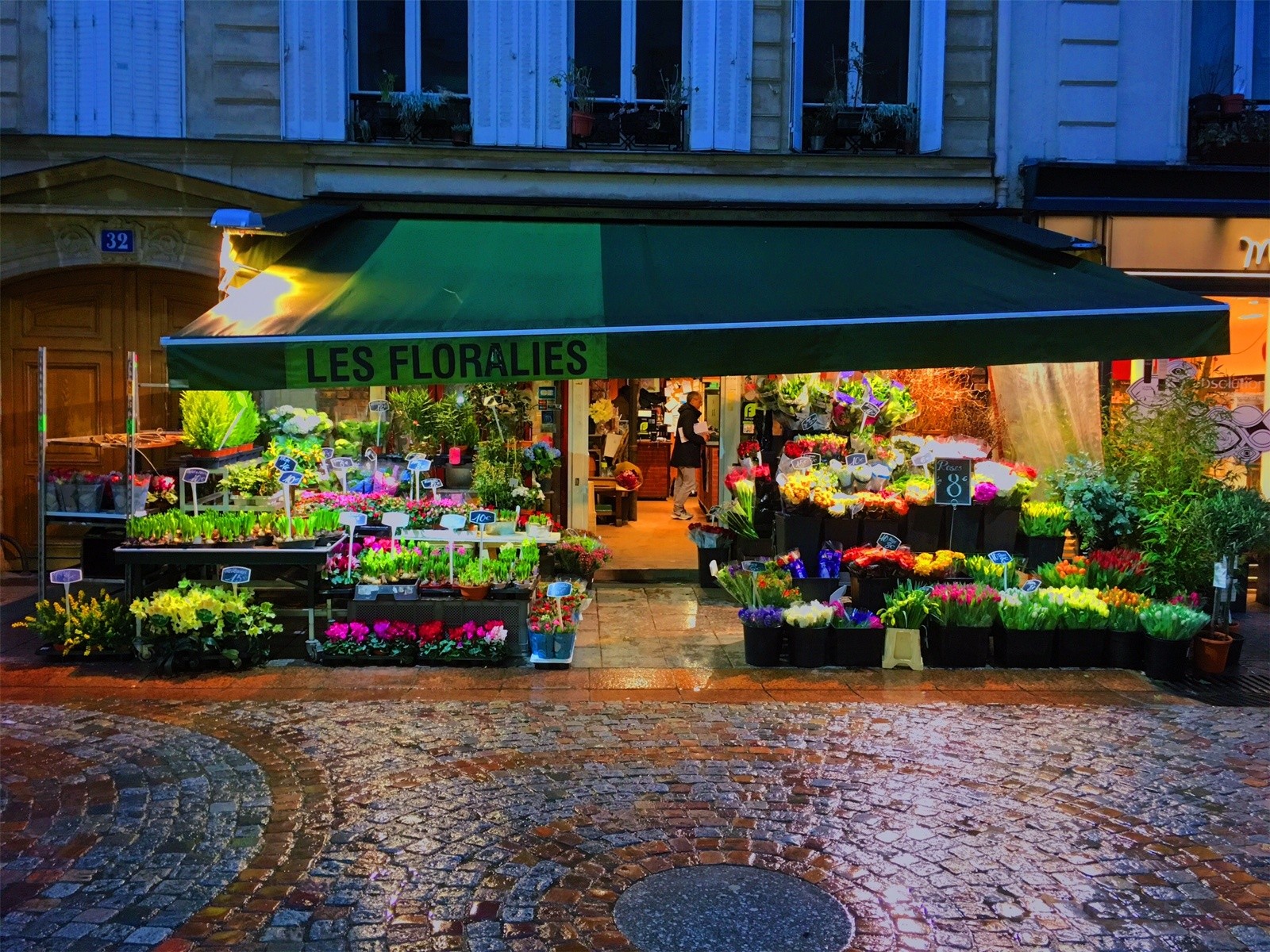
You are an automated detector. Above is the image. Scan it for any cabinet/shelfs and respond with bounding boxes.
[752,406,789,537]
[588,419,630,527]
[35,345,184,623]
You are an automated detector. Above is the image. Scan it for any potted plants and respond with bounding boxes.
[801,40,919,156]
[549,61,691,137]
[1188,43,1270,166]
[126,379,562,549]
[1040,354,1270,688]
[344,69,472,149]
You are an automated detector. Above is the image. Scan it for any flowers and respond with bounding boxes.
[606,93,640,120]
[7,395,642,660]
[685,370,1213,639]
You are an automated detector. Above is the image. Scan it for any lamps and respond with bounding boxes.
[206,208,266,231]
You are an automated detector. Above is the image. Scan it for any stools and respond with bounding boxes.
[593,486,622,528]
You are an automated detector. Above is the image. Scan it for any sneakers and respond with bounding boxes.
[671,506,693,520]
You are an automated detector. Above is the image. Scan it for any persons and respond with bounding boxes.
[670,392,709,521]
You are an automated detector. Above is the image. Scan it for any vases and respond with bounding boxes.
[1014,528,1067,570]
[34,641,252,676]
[488,587,534,602]
[938,506,984,553]
[898,504,945,553]
[457,583,491,600]
[695,546,730,591]
[431,521,447,530]
[848,574,891,619]
[621,112,640,136]
[881,508,902,522]
[832,428,851,450]
[791,429,808,443]
[353,576,422,601]
[978,504,1021,555]
[318,588,356,610]
[862,519,899,548]
[418,578,461,598]
[740,624,1191,685]
[876,426,892,439]
[321,649,512,669]
[821,517,859,552]
[468,523,478,531]
[735,538,773,569]
[775,511,823,578]
[813,429,830,436]
[792,577,839,606]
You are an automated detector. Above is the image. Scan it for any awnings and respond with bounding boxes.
[162,220,1230,392]
